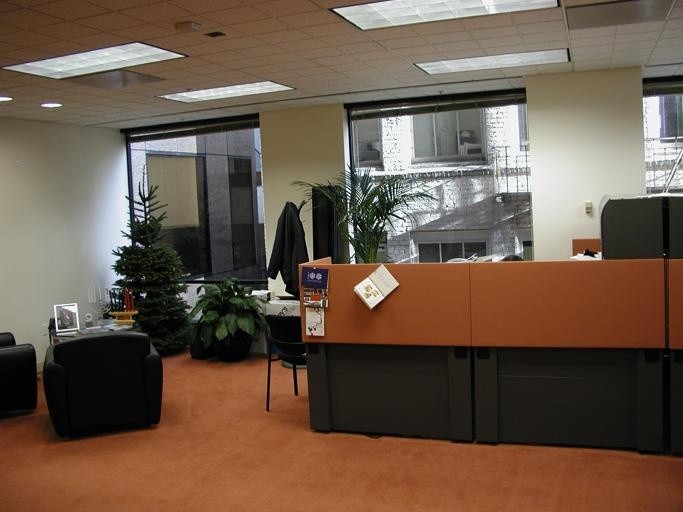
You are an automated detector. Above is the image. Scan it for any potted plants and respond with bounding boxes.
[186,273,270,362]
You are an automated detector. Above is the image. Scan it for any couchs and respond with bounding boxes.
[41,331,162,440]
[0,331,36,416]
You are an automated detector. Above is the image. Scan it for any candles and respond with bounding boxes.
[86,286,135,313]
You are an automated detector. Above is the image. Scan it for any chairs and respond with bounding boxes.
[260,315,308,411]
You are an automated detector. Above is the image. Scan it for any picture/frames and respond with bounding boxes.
[53,301,79,333]
